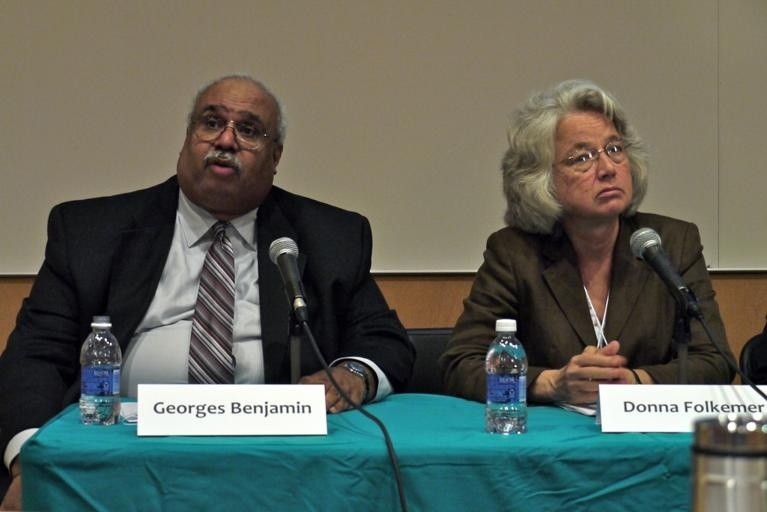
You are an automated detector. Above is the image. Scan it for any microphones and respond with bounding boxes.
[628,227,702,320]
[267,237,310,324]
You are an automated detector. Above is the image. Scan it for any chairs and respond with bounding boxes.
[394,326,463,393]
[739,329,763,383]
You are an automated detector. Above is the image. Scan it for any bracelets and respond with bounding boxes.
[629,366,642,384]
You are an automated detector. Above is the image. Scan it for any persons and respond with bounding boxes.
[440,77,738,404]
[0,74,417,512]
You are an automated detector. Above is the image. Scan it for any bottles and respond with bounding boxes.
[78,314,119,426]
[486,319,527,432]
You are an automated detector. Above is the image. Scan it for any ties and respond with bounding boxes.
[189,221,235,384]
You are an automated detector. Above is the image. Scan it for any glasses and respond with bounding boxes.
[195,115,270,148]
[561,142,626,171]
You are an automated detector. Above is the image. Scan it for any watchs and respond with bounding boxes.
[338,361,370,404]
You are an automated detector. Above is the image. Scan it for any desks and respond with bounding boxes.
[19,392,696,510]
